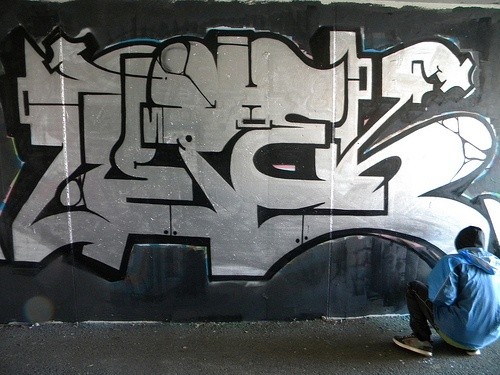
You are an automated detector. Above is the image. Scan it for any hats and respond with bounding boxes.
[455,226,485,250]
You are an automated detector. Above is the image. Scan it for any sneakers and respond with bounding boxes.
[467,349,480,355]
[392,334,433,356]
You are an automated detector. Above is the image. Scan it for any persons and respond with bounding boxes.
[393,225,500,356]
